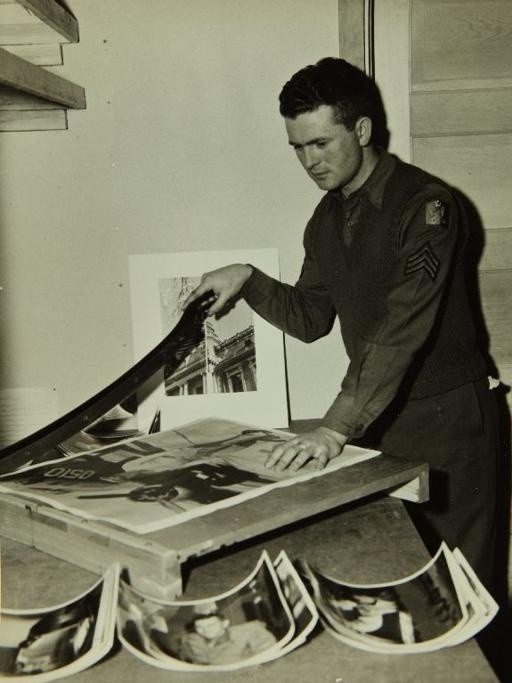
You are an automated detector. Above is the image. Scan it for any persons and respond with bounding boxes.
[127,464,256,503]
[12,615,93,676]
[322,587,416,646]
[173,612,280,666]
[181,56,512,682]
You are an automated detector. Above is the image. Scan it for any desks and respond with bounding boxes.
[1,420,501,683]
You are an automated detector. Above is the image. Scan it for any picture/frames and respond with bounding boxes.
[128,247,291,433]
